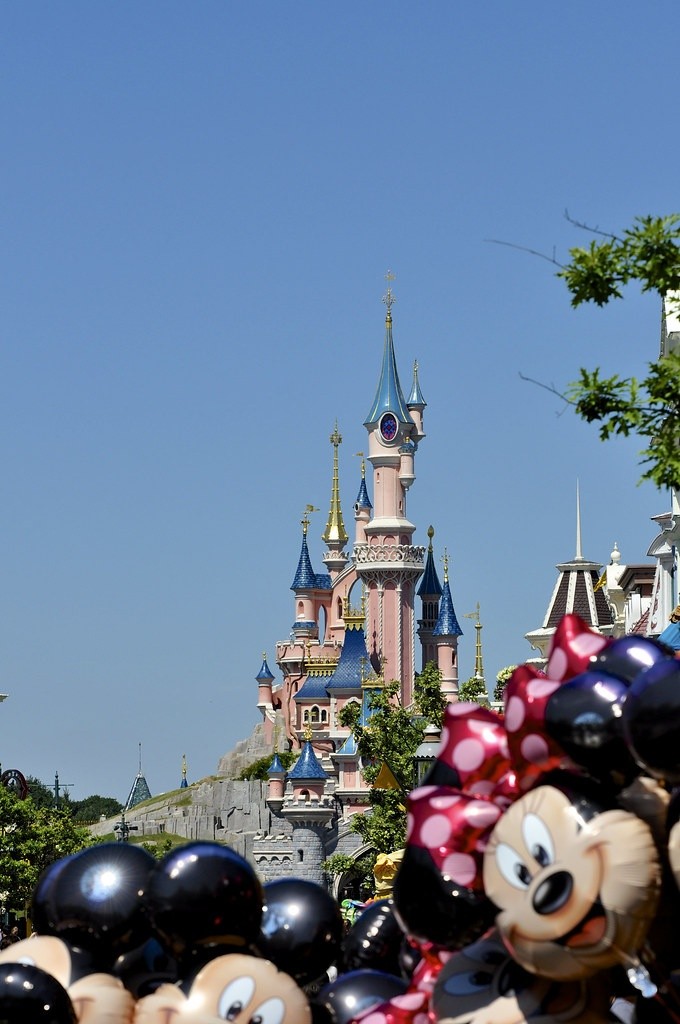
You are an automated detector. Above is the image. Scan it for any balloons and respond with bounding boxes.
[1,614,680,1024]
